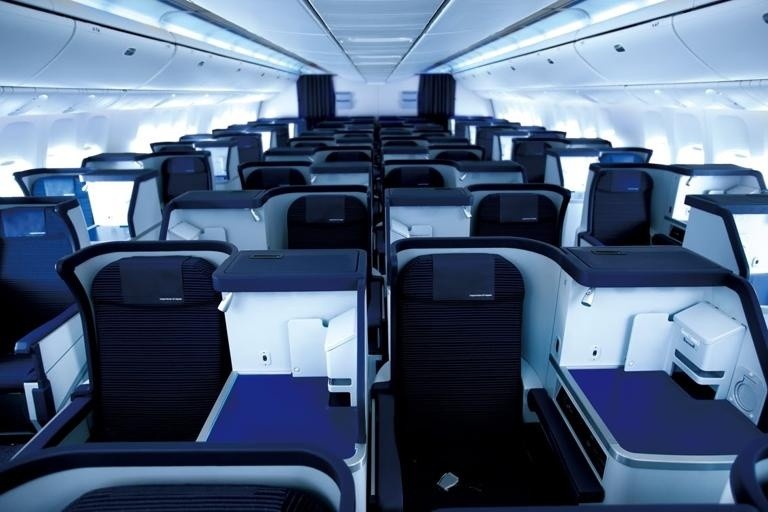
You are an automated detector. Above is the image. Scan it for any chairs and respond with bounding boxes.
[0,112,767,512]
